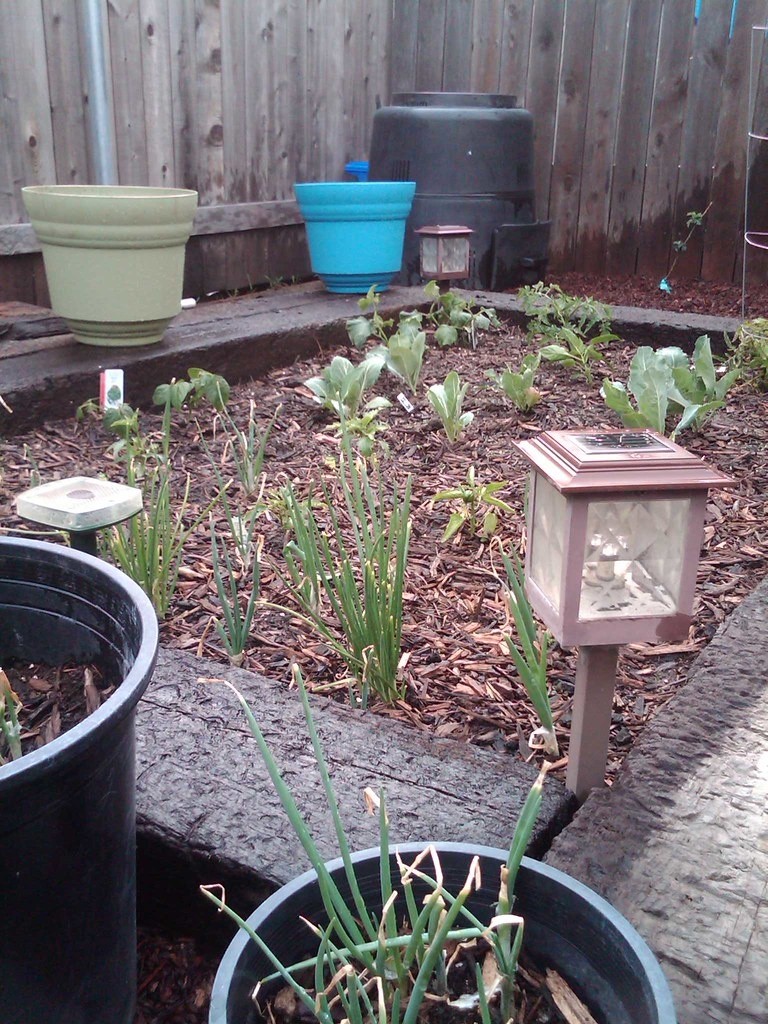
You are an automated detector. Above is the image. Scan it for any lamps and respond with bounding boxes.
[414,224,473,296]
[507,427,735,803]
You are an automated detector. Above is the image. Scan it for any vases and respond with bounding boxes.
[0,536,158,1024]
[21,185,198,347]
[294,181,416,295]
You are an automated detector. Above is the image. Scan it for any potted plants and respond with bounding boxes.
[199,661,678,1024]
